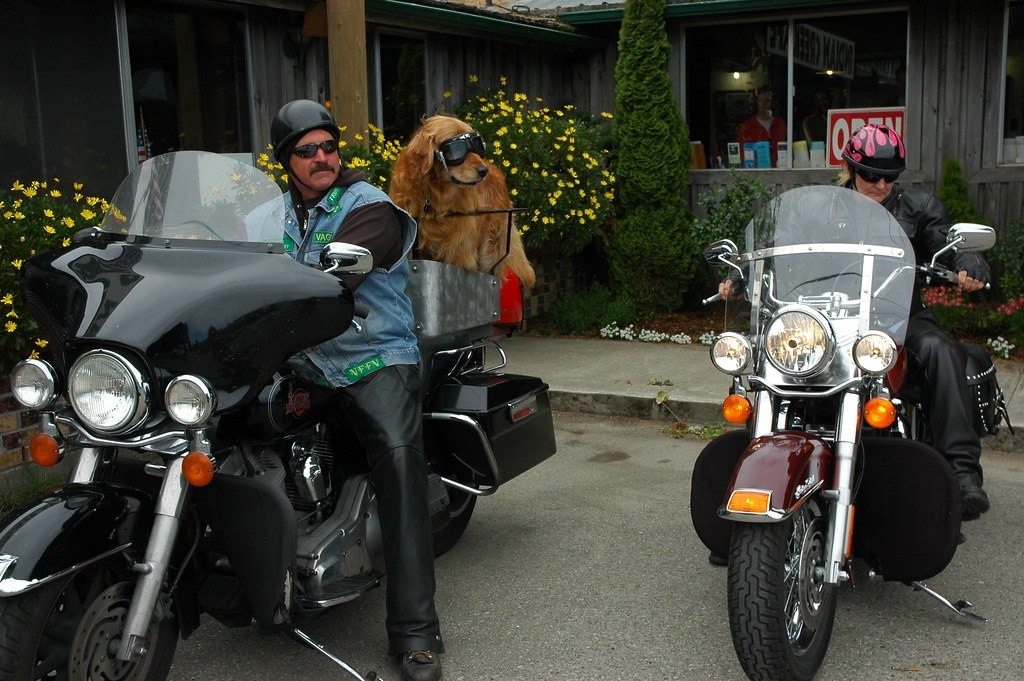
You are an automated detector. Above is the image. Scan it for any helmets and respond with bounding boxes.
[270,99,341,160]
[841,124,906,173]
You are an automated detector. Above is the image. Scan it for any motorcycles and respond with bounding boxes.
[688,186,1007,681]
[0,151,557,680]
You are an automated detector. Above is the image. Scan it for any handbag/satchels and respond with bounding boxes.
[960,342,1017,437]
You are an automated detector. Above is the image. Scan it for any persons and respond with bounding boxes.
[736,86,787,168]
[229,100,445,681]
[720,123,991,522]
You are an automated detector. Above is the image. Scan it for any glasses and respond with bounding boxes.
[282,139,339,159]
[853,166,899,183]
[431,131,486,168]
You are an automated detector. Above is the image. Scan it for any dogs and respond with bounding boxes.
[387,114,537,290]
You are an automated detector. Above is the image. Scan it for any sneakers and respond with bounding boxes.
[949,469,989,521]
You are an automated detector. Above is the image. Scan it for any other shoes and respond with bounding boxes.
[401,649,441,681]
